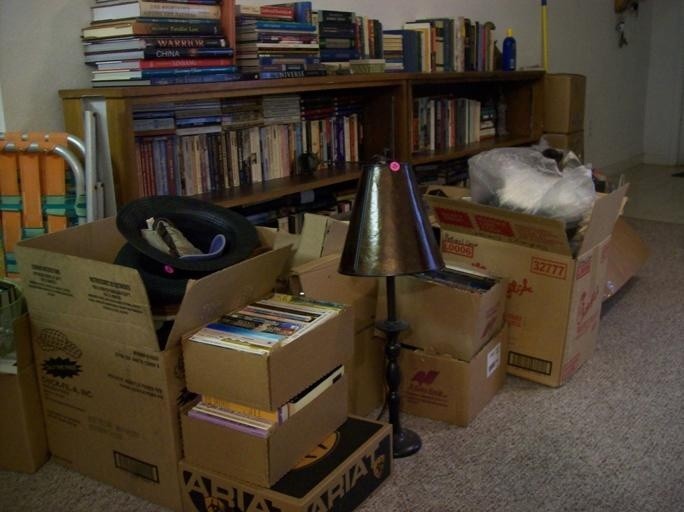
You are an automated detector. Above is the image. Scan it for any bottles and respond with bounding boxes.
[502,28,516,72]
[497,94,510,138]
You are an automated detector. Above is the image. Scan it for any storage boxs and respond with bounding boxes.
[1,70,636,511]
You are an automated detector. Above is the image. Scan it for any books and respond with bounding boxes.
[132,91,496,196]
[79,1,494,89]
[186,294,348,439]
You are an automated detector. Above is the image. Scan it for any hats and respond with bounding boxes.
[112,194,261,303]
